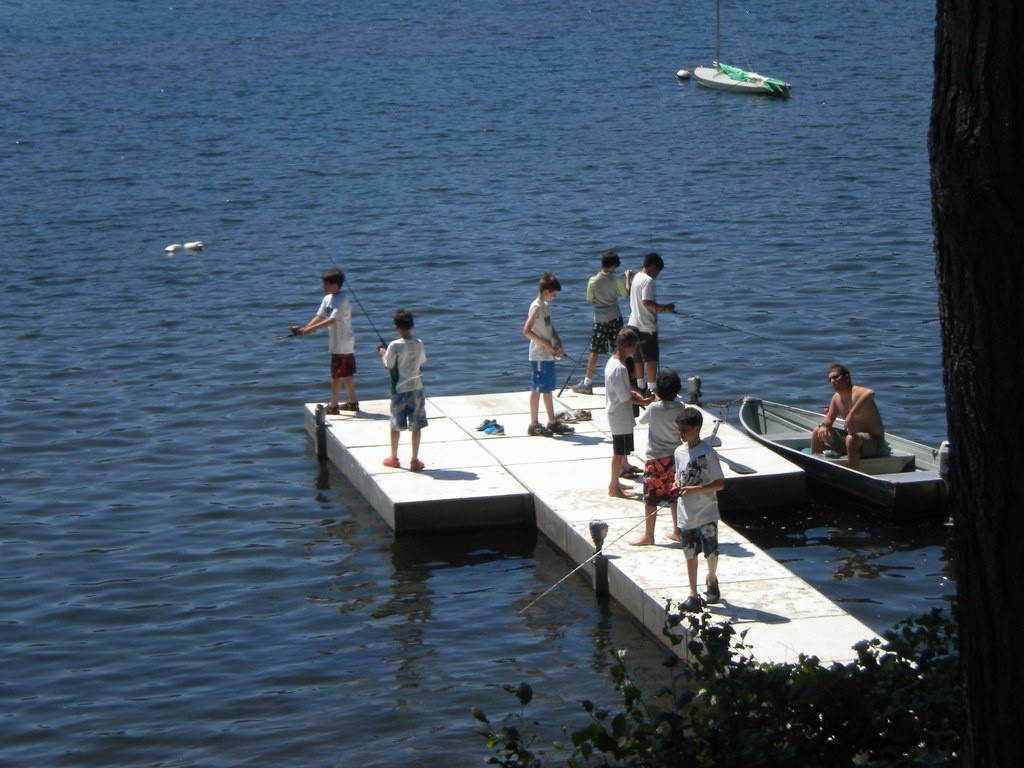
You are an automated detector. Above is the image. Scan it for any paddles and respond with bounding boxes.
[718,454,758,474]
[702,418,724,448]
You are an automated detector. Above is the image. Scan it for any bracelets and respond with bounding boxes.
[818,423,823,427]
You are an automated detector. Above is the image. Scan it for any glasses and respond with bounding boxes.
[827,373,842,382]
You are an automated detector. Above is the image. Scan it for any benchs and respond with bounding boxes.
[817,451,915,475]
[760,433,812,451]
[873,470,941,483]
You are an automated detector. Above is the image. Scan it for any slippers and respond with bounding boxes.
[630,465,643,473]
[619,470,639,478]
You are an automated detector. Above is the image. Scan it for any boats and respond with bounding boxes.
[695,0,793,96]
[739,396,950,522]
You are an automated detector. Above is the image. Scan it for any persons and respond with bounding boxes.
[627,253,674,398]
[628,369,685,545]
[523,273,575,436]
[379,309,428,471]
[673,407,725,611]
[298,269,360,414]
[811,364,885,470]
[604,325,655,498]
[572,251,632,394]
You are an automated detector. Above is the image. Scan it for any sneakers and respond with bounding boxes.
[485,424,504,435]
[548,421,575,434]
[528,423,552,436]
[572,379,592,394]
[475,419,496,430]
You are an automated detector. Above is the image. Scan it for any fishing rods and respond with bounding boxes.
[557,273,625,398]
[266,325,303,342]
[518,478,704,615]
[551,348,602,378]
[308,224,389,351]
[669,300,773,340]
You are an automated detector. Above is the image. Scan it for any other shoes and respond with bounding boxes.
[325,404,339,414]
[383,458,400,467]
[339,400,359,410]
[706,573,720,602]
[555,410,577,423]
[679,594,702,611]
[574,410,591,421]
[410,460,424,470]
